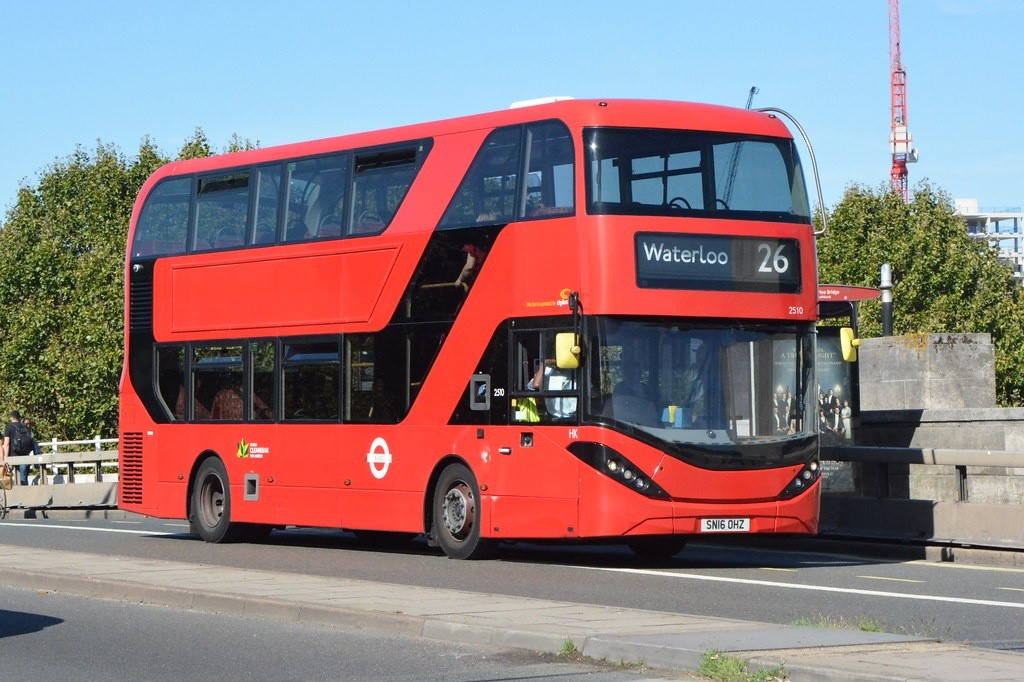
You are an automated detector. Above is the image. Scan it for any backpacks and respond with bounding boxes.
[10,423,33,455]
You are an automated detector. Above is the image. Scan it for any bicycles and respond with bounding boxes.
[0,463,11,519]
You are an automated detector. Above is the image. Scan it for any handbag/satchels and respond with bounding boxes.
[0,462,12,490]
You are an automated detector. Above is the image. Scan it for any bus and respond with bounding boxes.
[116,95,864,561]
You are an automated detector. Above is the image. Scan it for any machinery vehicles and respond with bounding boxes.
[715,85,762,210]
[885,1,919,205]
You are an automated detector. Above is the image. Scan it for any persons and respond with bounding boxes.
[773,380,852,436]
[527,345,598,422]
[612,360,651,401]
[20,418,34,440]
[3,410,33,486]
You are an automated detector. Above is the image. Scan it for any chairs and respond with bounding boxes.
[144,209,385,256]
